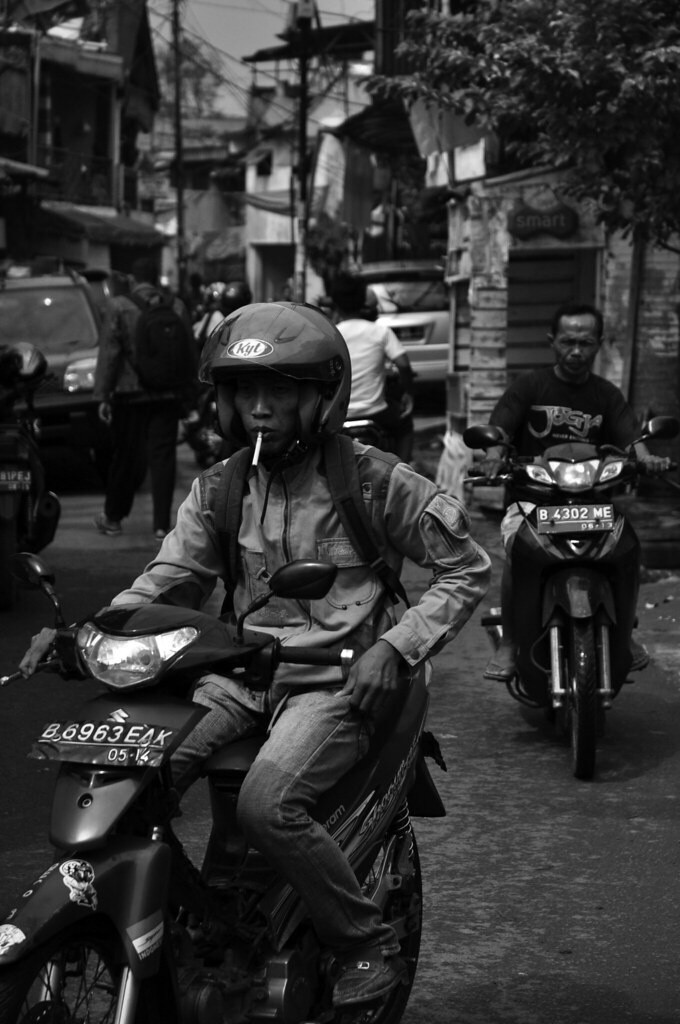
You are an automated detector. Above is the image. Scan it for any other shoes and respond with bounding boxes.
[155,528,167,540]
[92,512,121,534]
[333,955,410,1005]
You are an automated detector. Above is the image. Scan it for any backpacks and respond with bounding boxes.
[117,288,196,398]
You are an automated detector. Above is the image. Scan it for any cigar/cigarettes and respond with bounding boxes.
[252,429,262,464]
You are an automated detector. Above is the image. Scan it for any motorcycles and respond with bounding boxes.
[0,341,62,588]
[459,412,680,779]
[2,488,451,1024]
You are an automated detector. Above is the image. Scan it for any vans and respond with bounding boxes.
[352,260,452,382]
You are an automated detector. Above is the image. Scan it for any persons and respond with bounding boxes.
[18,299,493,1004]
[468,304,671,683]
[91,258,418,540]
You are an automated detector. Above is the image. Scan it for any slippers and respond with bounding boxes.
[483,655,518,681]
[618,641,650,672]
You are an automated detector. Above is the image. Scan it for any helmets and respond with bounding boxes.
[197,301,353,444]
[202,281,251,312]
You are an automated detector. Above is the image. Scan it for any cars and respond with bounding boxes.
[1,252,134,472]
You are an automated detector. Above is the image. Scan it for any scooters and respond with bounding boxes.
[338,365,425,460]
